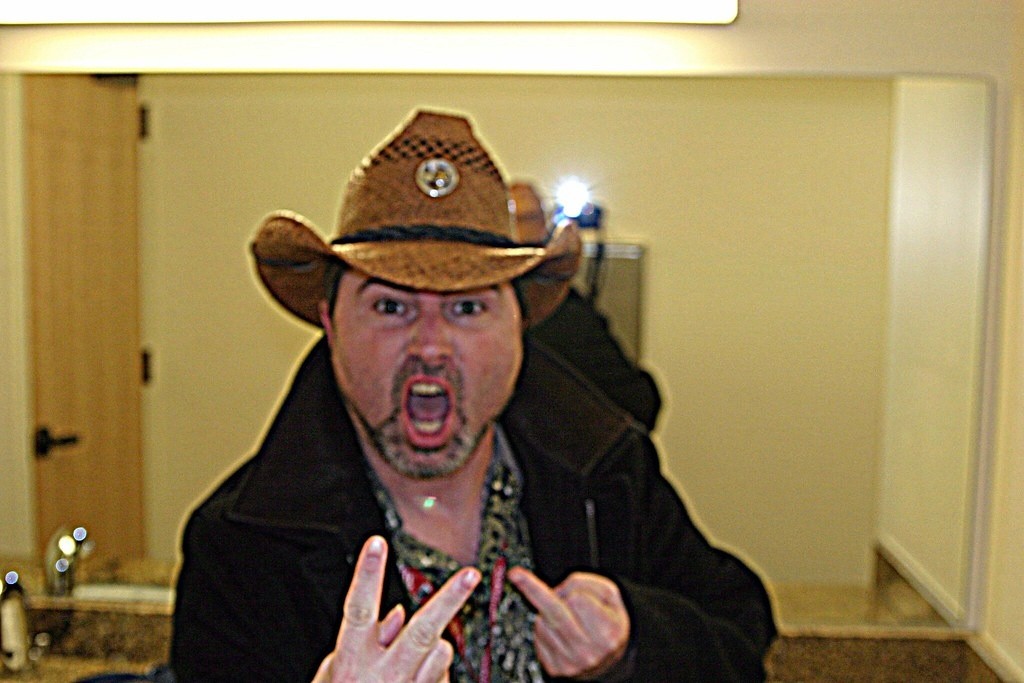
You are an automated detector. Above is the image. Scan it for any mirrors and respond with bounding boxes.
[1,73,1001,634]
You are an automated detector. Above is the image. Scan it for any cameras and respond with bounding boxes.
[554,200,603,229]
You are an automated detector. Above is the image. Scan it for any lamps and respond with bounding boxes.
[2,0,739,25]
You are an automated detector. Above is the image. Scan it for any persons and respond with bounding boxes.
[170,111,778,683]
[511,184,663,433]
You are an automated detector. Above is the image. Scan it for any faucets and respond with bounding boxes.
[40,526,83,598]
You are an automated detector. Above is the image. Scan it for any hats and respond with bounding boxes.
[250,109,582,331]
[509,184,552,242]
